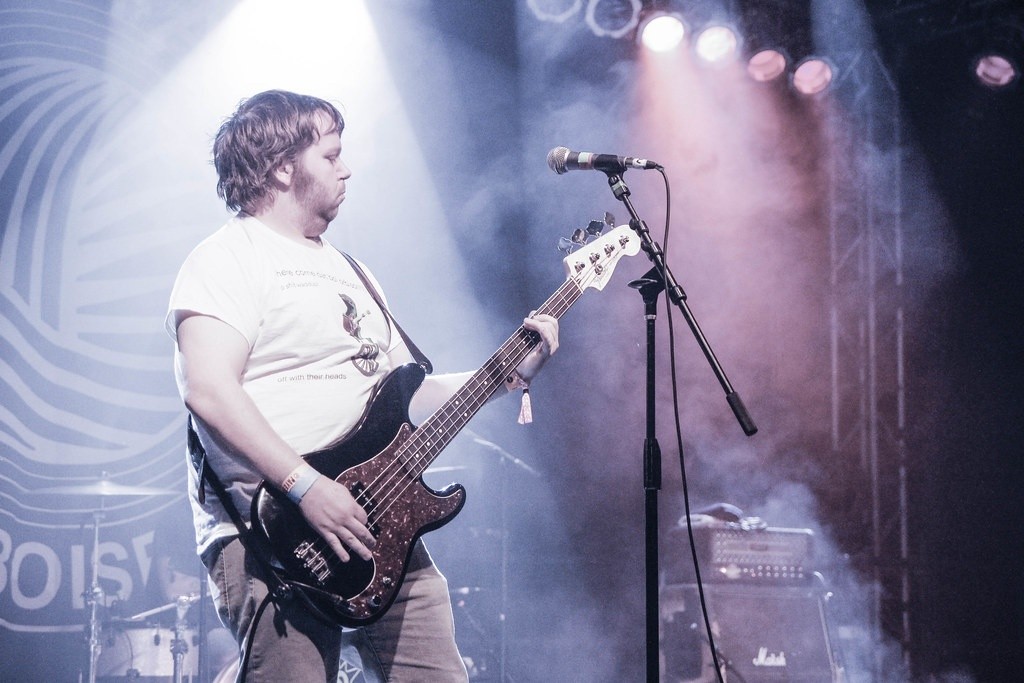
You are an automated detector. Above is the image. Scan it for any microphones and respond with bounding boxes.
[547,147,656,174]
[452,587,482,596]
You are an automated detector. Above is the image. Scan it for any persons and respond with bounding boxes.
[162,88,560,683]
[147,499,240,683]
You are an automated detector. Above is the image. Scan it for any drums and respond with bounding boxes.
[210,649,365,683]
[254,209,650,630]
[95,618,201,677]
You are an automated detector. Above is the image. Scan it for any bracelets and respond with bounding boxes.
[500,365,540,427]
[279,460,322,503]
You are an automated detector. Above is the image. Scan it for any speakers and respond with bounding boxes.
[660,583,837,683]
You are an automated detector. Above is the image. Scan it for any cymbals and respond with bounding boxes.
[449,585,487,597]
[420,464,467,474]
[24,477,180,496]
